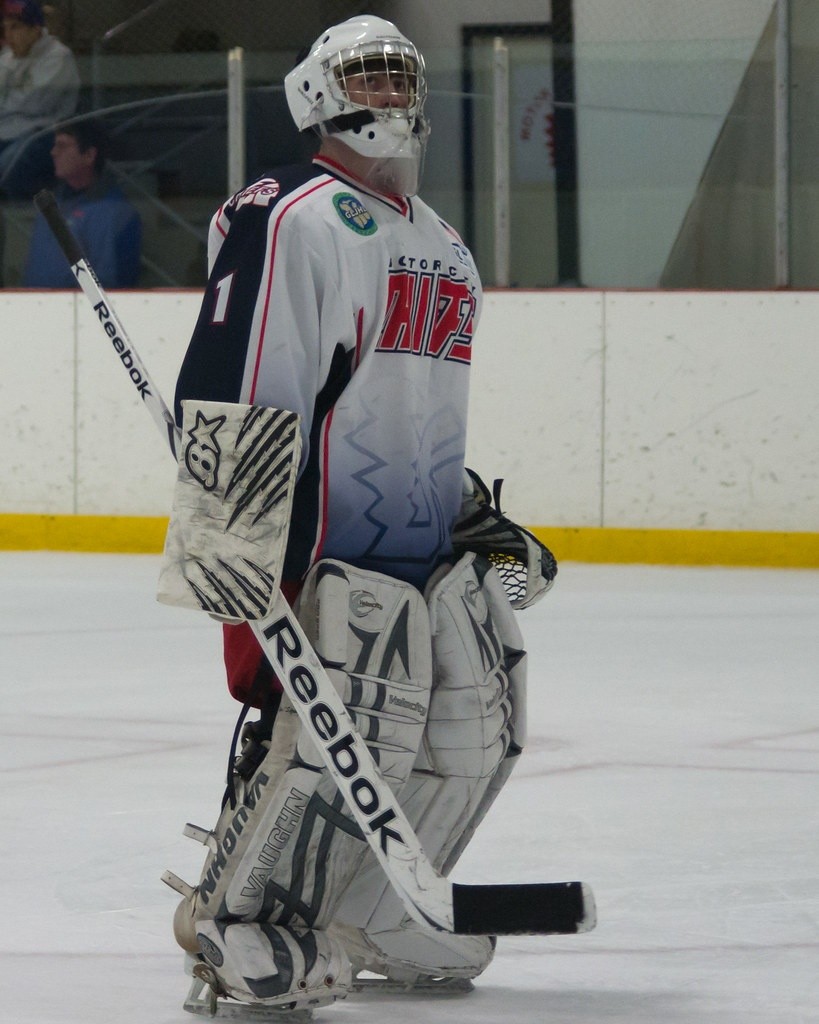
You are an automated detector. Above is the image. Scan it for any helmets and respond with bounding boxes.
[283,15,426,159]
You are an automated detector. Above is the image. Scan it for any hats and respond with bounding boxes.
[0,0,46,27]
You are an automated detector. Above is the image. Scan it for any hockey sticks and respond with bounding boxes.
[31,186,600,940]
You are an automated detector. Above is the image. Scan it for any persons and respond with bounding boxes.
[156,13,560,1023]
[0,0,83,202]
[23,114,143,289]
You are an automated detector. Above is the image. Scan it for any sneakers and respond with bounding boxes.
[331,921,475,998]
[173,896,315,1024]
